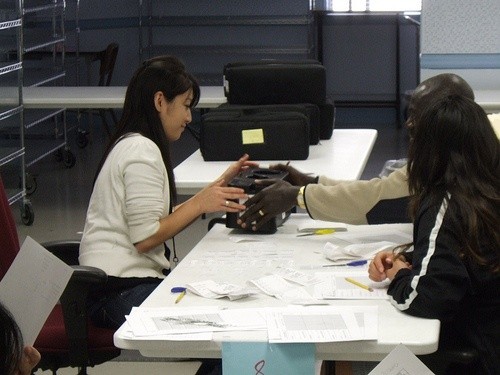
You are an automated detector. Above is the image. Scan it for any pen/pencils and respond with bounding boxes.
[344,276,373,291]
[393,248,407,261]
[175,291,186,304]
[297,227,347,232]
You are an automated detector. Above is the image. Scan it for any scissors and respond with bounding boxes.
[322,260,367,267]
[171,287,186,292]
[296,230,335,237]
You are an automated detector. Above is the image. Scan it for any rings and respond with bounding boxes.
[226,201,229,206]
[259,210,264,216]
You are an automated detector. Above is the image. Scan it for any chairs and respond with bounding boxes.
[0,172,122,375]
[66,43,119,137]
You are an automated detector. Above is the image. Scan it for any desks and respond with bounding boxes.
[173,129,378,219]
[0,86,227,141]
[3,41,110,145]
[114,213,441,375]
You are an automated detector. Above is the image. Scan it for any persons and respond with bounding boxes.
[0,303,41,375]
[237,73,473,232]
[78,57,258,375]
[322,94,500,375]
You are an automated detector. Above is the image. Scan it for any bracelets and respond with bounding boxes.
[297,186,306,210]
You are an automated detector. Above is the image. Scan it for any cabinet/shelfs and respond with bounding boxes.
[0,0,319,226]
